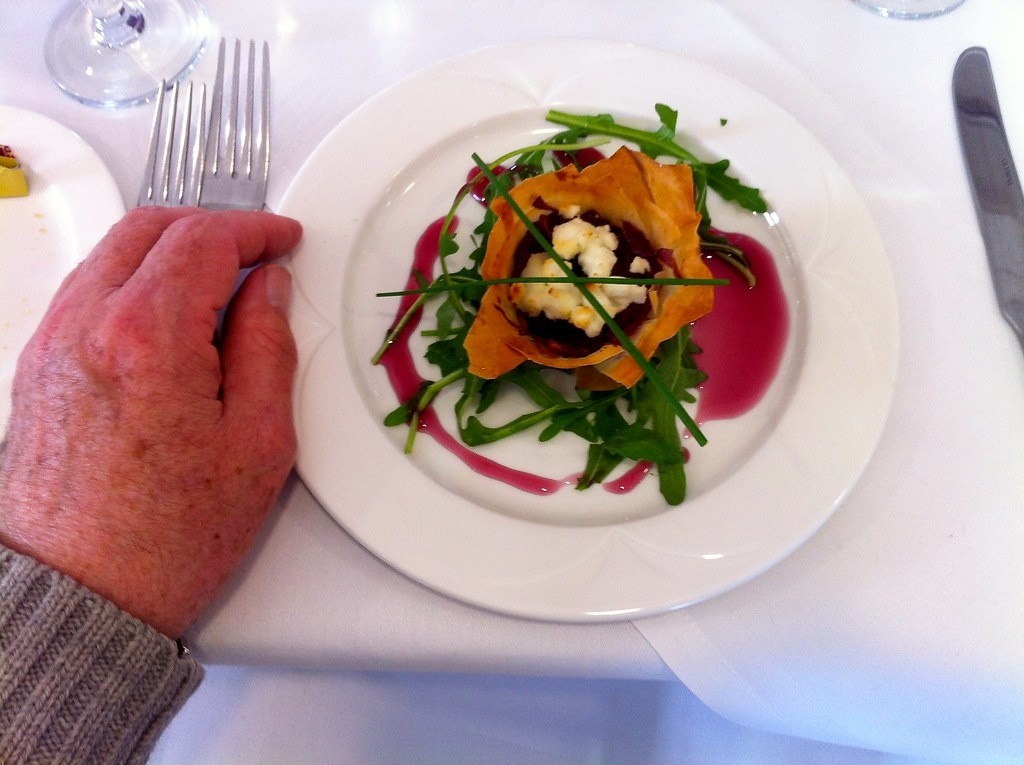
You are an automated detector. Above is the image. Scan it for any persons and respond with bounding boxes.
[0,206,302,765]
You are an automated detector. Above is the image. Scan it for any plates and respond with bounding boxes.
[1,104,127,444]
[252,37,900,625]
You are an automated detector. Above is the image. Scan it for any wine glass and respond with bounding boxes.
[44,0,209,108]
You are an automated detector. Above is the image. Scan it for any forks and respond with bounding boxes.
[136,78,208,207]
[199,36,270,214]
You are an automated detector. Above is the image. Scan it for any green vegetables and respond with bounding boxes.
[369,102,769,511]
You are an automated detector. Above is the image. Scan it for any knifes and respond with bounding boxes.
[951,46,1024,355]
